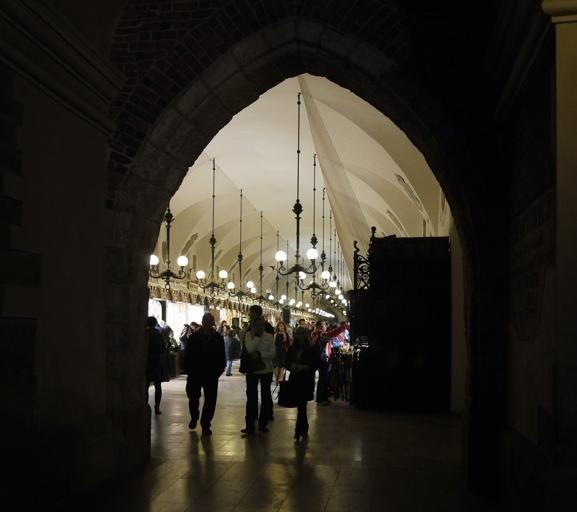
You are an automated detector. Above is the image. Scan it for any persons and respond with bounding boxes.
[183,313,226,428]
[145,305,349,416]
[283,326,323,442]
[240,316,277,435]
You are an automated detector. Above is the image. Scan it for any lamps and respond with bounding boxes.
[148,92,349,324]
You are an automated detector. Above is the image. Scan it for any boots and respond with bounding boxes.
[153,404,162,414]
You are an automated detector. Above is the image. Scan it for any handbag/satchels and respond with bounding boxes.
[238,329,265,373]
[275,378,298,408]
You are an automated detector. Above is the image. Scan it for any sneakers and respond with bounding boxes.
[187,412,213,435]
[317,399,330,405]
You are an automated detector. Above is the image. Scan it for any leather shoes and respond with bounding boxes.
[240,426,272,434]
